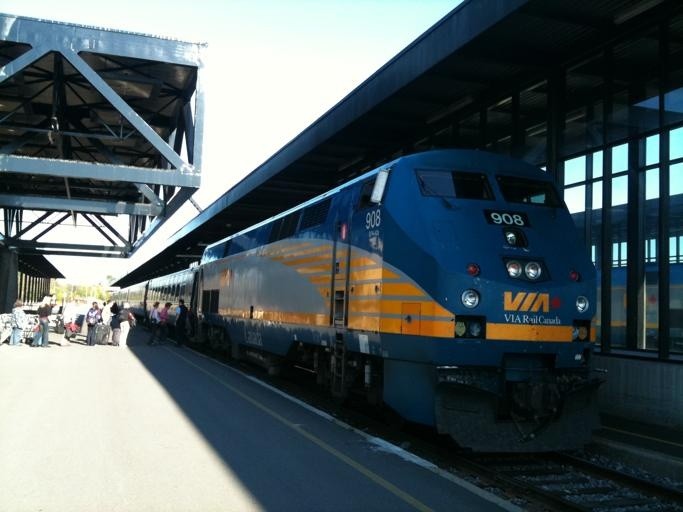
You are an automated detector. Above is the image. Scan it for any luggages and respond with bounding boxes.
[95,325,110,345]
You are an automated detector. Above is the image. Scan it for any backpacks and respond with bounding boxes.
[87,309,99,326]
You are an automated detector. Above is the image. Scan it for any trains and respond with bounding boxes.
[105,148,612,454]
[591,262,683,350]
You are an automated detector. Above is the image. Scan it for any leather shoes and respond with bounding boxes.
[41,343,50,347]
[32,344,41,348]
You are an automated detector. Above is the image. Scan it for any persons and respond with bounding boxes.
[0,292,190,349]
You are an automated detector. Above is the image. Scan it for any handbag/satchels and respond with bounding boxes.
[66,323,79,338]
[150,319,161,329]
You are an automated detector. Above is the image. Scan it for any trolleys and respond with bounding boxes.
[6,314,38,343]
